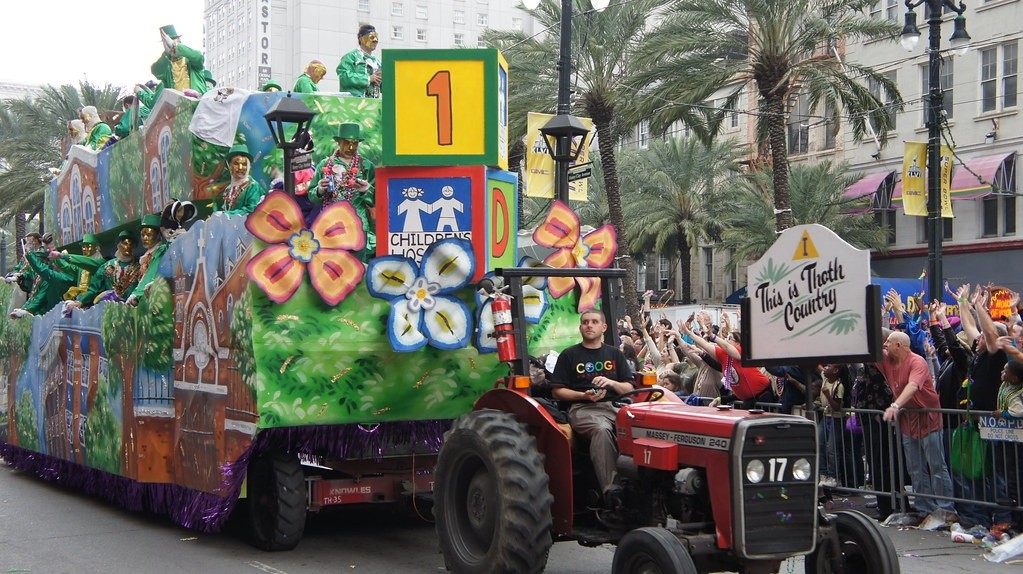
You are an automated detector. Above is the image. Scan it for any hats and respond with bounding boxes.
[204,70,216,87]
[227,145,253,162]
[141,214,161,227]
[333,123,365,142]
[79,233,102,247]
[118,230,138,247]
[264,80,282,92]
[159,25,182,42]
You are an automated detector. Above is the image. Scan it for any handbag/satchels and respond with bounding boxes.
[950,417,986,480]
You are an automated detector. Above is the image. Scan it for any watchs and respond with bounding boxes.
[891,403,898,408]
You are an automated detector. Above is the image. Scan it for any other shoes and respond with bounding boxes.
[601,506,625,525]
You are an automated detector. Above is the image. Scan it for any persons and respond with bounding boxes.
[220,144,260,216]
[308,124,375,233]
[4,196,198,319]
[262,59,326,91]
[551,309,638,506]
[51,25,217,176]
[336,23,383,98]
[616,280,1023,529]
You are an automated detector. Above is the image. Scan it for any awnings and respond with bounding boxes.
[835,168,897,216]
[947,150,1018,200]
[889,165,931,208]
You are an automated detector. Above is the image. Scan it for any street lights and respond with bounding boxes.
[899,0,973,305]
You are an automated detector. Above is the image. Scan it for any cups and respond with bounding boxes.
[950,531,973,544]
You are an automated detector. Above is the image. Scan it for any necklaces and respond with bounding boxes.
[775,376,782,392]
[321,149,358,211]
[223,179,250,210]
[114,260,134,288]
[825,378,835,433]
[724,341,740,391]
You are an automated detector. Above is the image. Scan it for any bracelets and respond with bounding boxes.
[688,333,692,336]
[1011,312,1018,315]
[959,297,969,302]
[929,320,937,322]
[173,41,176,54]
[950,292,953,295]
[710,335,716,341]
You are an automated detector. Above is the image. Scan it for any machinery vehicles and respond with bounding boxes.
[0,49,901,574]
